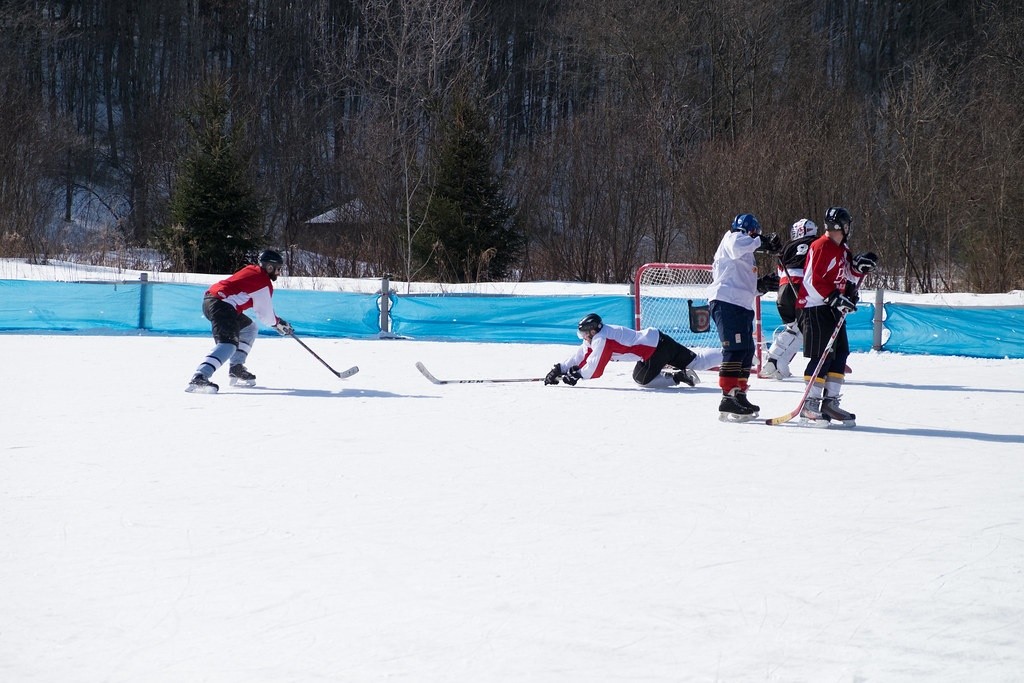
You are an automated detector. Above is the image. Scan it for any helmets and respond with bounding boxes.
[579,313,602,334]
[791,219,819,239]
[825,207,852,230]
[257,249,282,282]
[731,214,759,236]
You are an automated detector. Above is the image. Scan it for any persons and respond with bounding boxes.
[544,313,761,389]
[185,250,294,394]
[705,213,785,423]
[797,206,877,428]
[764,217,820,380]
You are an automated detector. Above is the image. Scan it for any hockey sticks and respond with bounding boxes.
[416,361,564,384]
[765,271,868,425]
[778,255,799,298]
[290,332,360,380]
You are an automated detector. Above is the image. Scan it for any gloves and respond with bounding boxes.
[274,318,295,336]
[756,271,781,294]
[823,290,857,323]
[759,232,782,254]
[544,363,561,385]
[852,253,878,275]
[563,365,584,386]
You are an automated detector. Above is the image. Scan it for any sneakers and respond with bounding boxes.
[797,398,830,428]
[822,389,857,427]
[672,367,701,388]
[719,391,753,422]
[734,388,760,418]
[229,362,256,387]
[186,372,219,394]
